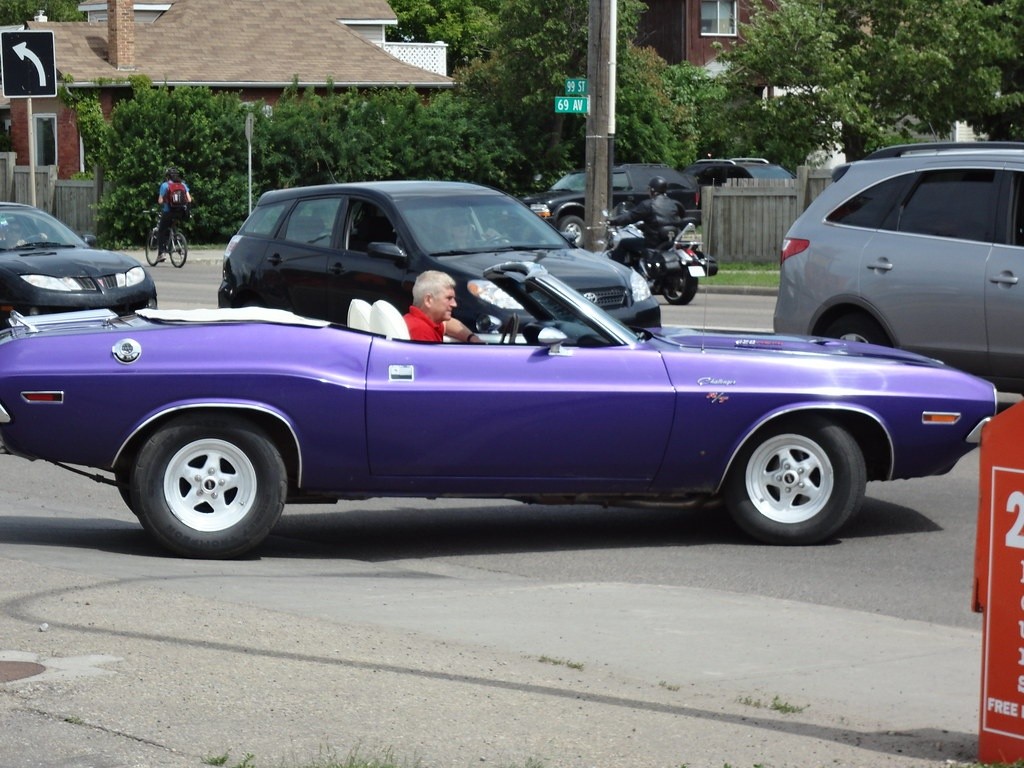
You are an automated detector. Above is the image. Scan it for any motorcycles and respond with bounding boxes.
[587,201,716,306]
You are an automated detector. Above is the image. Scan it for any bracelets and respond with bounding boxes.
[467,333,476,343]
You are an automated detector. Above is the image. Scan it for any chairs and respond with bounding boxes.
[345,295,414,344]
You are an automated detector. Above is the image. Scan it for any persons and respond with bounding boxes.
[607,177,685,265]
[155,167,192,263]
[0,219,47,248]
[443,210,501,250]
[402,269,489,345]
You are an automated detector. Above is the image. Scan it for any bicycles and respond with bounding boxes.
[141,209,189,268]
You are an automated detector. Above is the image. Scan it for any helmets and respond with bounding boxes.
[648,176,667,193]
[165,167,180,178]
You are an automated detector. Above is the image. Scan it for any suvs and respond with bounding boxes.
[520,166,703,249]
[772,140,1023,399]
[219,178,660,339]
[683,157,794,187]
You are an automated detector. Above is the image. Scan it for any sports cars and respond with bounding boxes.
[0,256,999,547]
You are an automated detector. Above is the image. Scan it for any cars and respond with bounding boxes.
[0,203,156,332]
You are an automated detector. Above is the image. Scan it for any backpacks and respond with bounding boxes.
[163,181,188,209]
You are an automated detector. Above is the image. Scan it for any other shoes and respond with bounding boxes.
[157,254,166,263]
